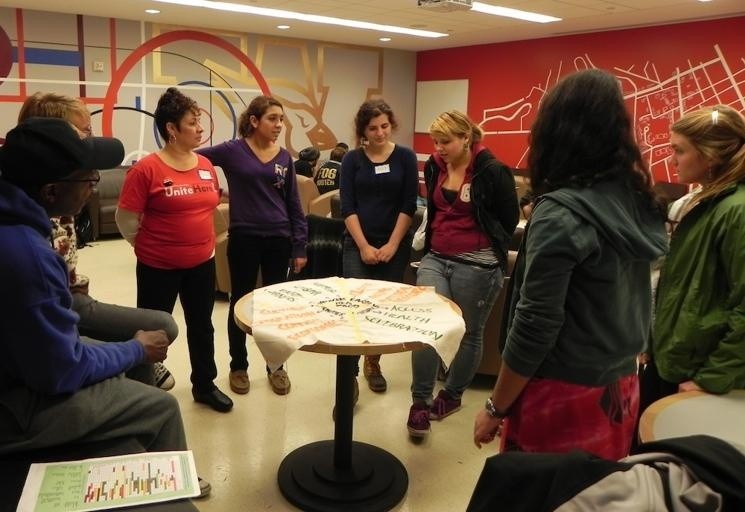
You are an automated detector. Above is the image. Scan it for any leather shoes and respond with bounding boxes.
[363,364,387,391]
[229,369,250,395]
[264,367,290,395]
[193,384,232,412]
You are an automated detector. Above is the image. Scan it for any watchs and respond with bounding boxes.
[484,396,511,420]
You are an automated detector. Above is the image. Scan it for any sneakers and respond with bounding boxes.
[152,362,175,391]
[428,391,463,420]
[407,402,431,437]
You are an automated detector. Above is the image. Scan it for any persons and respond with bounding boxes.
[332,97,420,421]
[17,90,179,392]
[335,143,348,152]
[295,146,321,178]
[113,86,234,413]
[405,108,520,439]
[628,104,744,454]
[186,97,314,396]
[315,147,347,196]
[520,186,537,219]
[473,67,673,465]
[0,120,210,502]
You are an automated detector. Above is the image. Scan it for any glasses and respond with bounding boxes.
[55,172,100,188]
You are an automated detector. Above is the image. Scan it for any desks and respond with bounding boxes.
[635,388,745,464]
[230,281,463,512]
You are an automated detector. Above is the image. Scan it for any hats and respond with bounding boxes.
[299,148,320,161]
[0,120,125,183]
